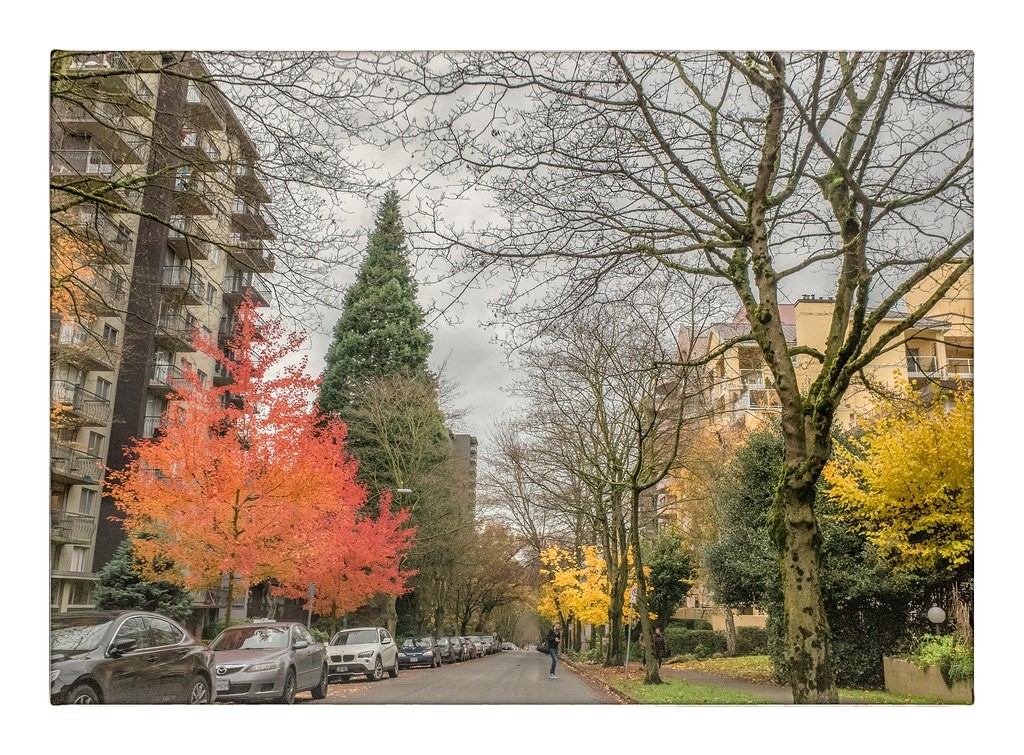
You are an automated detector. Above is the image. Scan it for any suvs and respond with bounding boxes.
[326,627,399,681]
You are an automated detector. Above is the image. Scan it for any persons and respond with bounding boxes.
[639,628,666,670]
[548,622,562,679]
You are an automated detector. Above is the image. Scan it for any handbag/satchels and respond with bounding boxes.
[537,629,552,654]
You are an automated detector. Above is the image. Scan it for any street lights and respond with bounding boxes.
[343,488,412,628]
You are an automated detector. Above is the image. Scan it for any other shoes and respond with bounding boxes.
[549,673,558,679]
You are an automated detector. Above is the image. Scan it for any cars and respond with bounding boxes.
[50,609,216,704]
[398,634,501,669]
[208,622,329,703]
